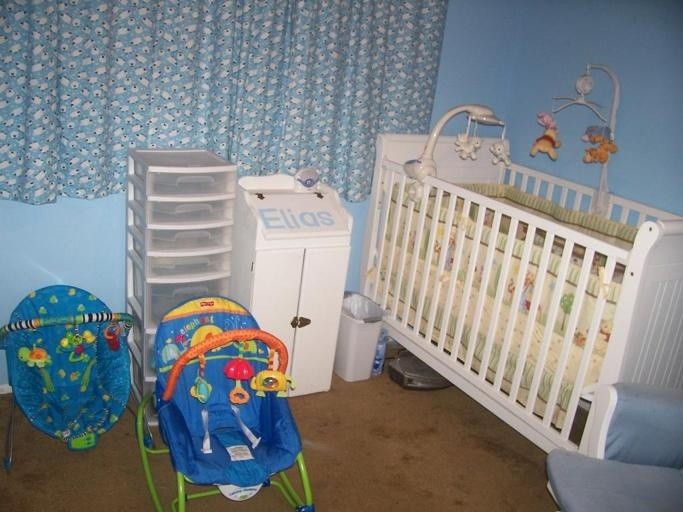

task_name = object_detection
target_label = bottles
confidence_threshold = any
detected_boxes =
[371,324,390,377]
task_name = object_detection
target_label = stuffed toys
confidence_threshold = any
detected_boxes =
[489,142,510,167]
[455,134,483,161]
[581,124,618,164]
[530,111,561,160]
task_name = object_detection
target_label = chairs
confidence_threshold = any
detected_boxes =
[544,381,683,512]
[132,295,314,512]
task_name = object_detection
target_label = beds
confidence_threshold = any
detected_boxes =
[372,129,683,512]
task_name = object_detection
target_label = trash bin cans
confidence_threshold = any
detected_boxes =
[335,293,388,383]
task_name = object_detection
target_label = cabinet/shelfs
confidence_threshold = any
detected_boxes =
[126,143,238,426]
